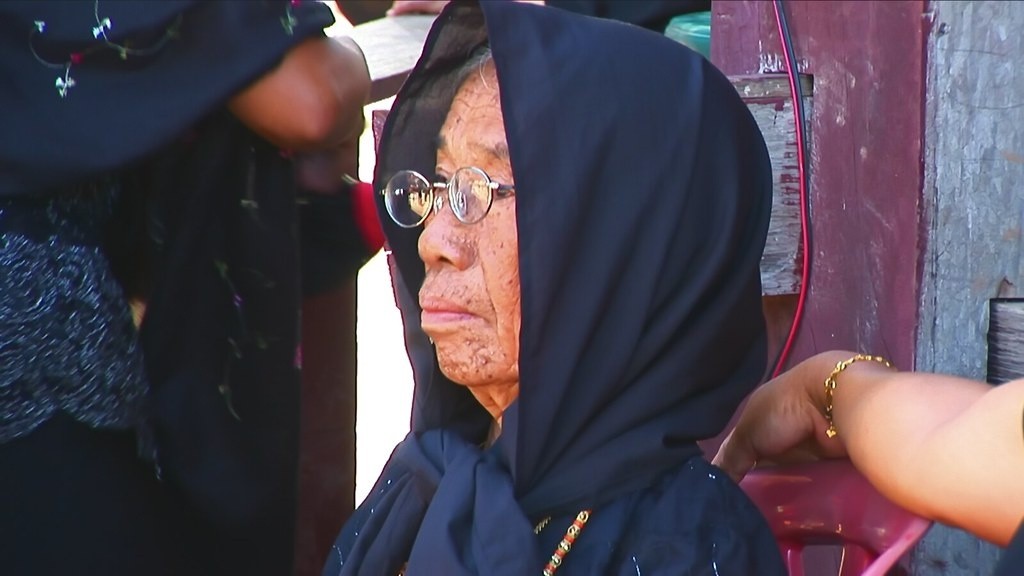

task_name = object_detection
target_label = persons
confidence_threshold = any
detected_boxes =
[320,0,785,576]
[0,0,373,575]
[387,0,710,101]
[709,269,1024,576]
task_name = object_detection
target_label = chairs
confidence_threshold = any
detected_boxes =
[737,455,934,576]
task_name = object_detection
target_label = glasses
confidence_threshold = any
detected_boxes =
[379,166,515,229]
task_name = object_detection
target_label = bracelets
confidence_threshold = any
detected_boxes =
[824,354,892,441]
